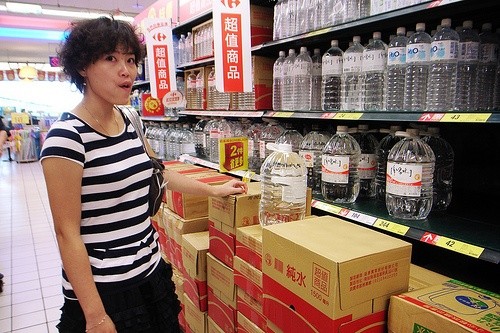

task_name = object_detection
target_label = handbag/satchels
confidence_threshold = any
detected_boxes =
[117,104,166,217]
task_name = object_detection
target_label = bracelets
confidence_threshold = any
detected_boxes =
[86,314,108,331]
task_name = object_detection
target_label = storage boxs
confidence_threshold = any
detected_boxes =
[153,154,500,333]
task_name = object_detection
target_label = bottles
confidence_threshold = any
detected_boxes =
[141,116,454,230]
[273,0,430,41]
[273,19,500,111]
[172,23,255,111]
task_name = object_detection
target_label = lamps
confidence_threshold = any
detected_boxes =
[18,63,38,78]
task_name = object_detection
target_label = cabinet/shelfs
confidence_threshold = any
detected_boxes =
[130,0,500,269]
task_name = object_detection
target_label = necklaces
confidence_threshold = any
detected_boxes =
[82,99,119,136]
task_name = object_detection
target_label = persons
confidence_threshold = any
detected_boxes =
[0,117,11,159]
[40,15,248,333]
[21,109,25,113]
